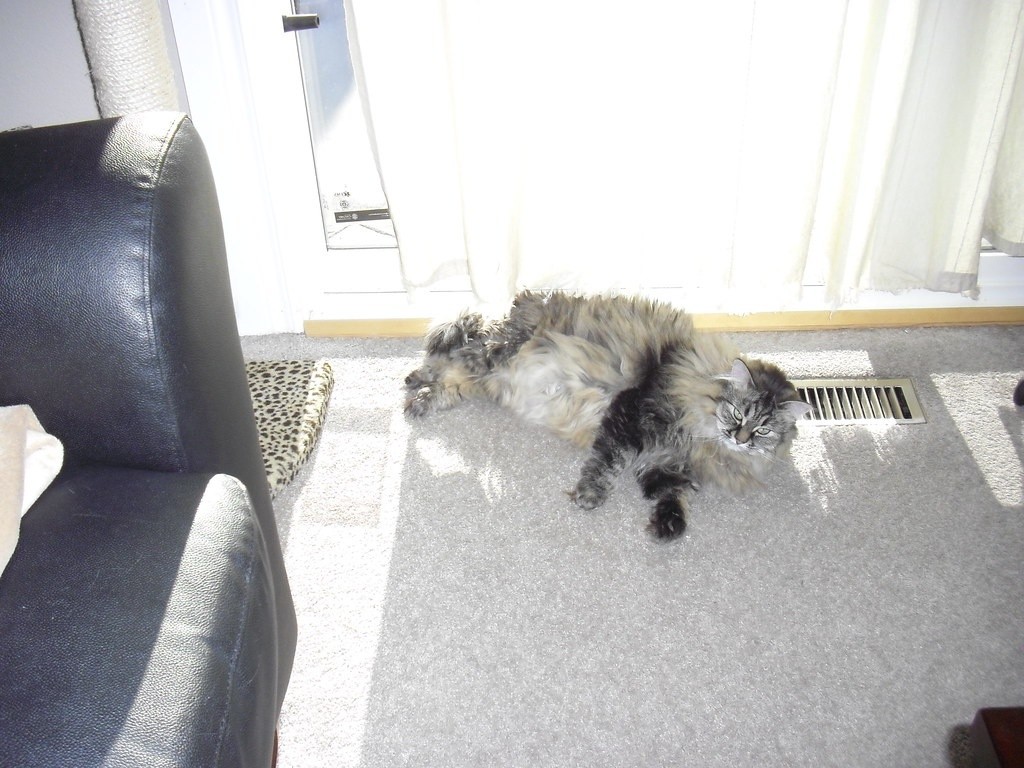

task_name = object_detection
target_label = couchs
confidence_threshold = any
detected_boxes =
[0,116,297,768]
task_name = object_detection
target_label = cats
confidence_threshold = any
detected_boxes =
[401,286,813,546]
